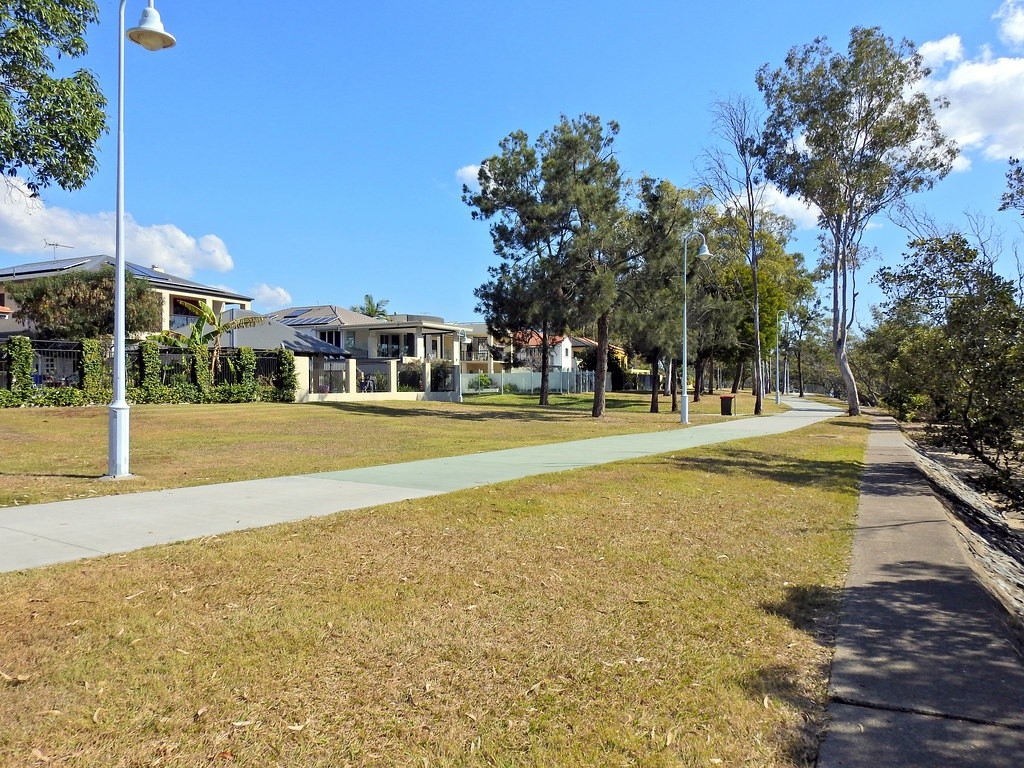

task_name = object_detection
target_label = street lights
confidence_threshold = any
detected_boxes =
[103,0,180,483]
[678,231,715,425]
[775,310,791,405]
